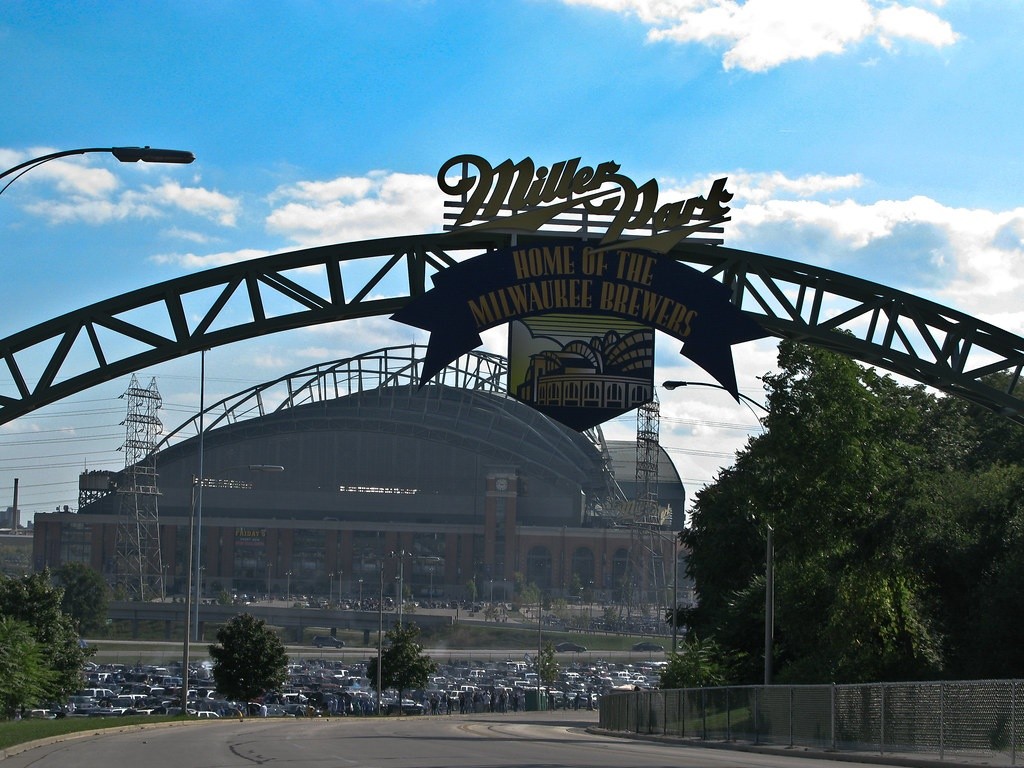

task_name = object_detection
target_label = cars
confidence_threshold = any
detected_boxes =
[555,642,587,653]
[198,593,488,612]
[633,641,665,653]
[30,655,669,719]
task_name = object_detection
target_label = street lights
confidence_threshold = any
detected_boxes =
[199,565,206,599]
[490,578,493,604]
[612,523,678,659]
[285,569,292,607]
[328,569,334,606]
[503,578,507,603]
[338,569,343,606]
[589,578,594,617]
[266,559,273,596]
[162,562,170,599]
[661,379,778,686]
[358,577,364,603]
[473,572,476,604]
[182,464,287,714]
[429,566,433,602]
[390,550,413,632]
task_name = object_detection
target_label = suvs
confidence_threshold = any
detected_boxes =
[312,635,346,650]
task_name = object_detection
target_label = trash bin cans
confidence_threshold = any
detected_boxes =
[525,688,548,711]
[549,690,566,709]
